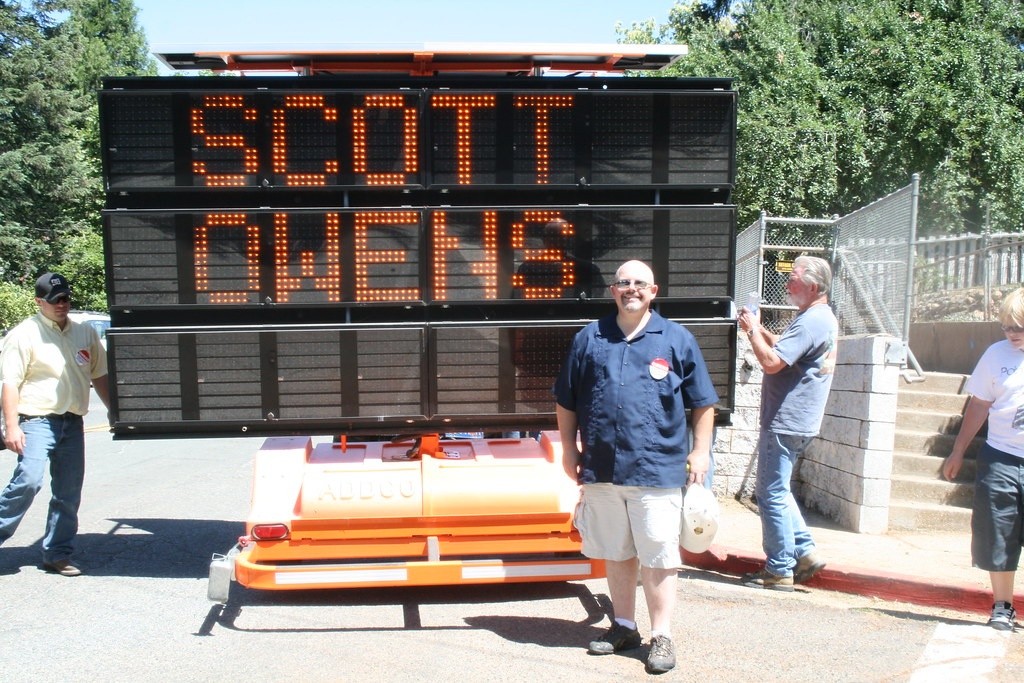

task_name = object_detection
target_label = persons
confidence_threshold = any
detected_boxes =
[0,273,116,576]
[554,259,719,673]
[944,286,1024,630]
[737,256,839,592]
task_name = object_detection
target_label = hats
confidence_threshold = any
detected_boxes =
[35,273,73,301]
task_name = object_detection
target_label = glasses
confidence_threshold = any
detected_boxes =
[1000,326,1024,332]
[45,294,71,304]
[613,279,652,289]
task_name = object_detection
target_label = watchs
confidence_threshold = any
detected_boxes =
[746,329,755,338]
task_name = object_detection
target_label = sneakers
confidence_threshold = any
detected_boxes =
[50,559,82,576]
[986,601,1016,630]
[589,622,641,654]
[647,636,676,671]
[742,569,794,592]
[793,553,826,582]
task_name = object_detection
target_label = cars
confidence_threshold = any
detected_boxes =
[66,309,111,343]
